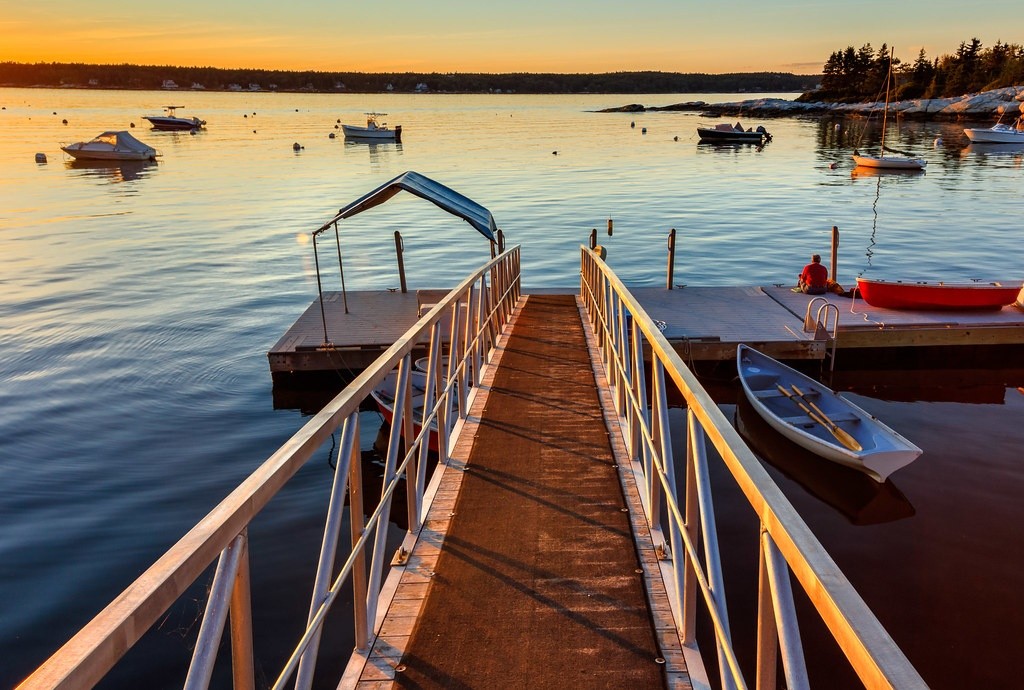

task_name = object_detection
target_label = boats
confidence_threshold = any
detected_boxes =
[141,107,206,131]
[61,130,163,160]
[963,123,1024,144]
[737,343,924,483]
[696,124,763,143]
[68,158,158,182]
[369,354,484,453]
[341,113,401,138]
[854,275,1024,310]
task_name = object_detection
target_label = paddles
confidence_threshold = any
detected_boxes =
[374,390,437,425]
[773,380,864,452]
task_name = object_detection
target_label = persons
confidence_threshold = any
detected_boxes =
[797,255,828,296]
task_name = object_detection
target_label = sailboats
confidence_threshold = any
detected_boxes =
[851,46,928,169]
